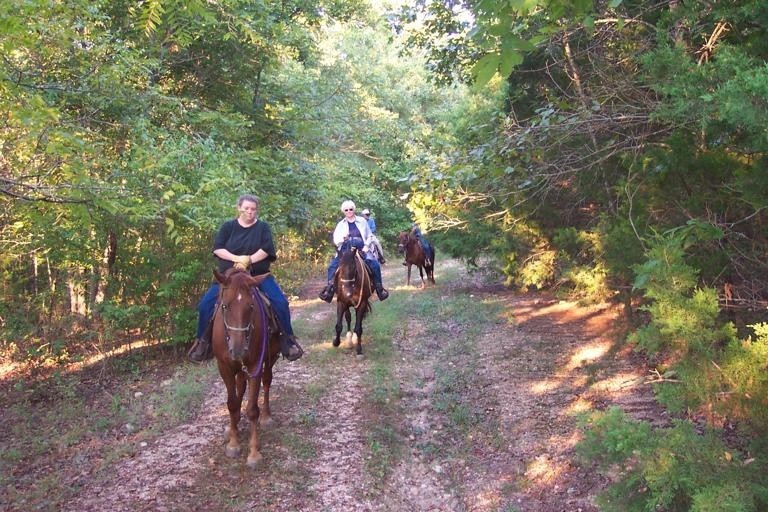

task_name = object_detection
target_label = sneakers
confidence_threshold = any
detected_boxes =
[279,331,304,362]
[322,285,335,304]
[190,339,214,361]
[376,287,389,301]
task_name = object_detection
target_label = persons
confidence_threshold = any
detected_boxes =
[190,193,302,364]
[320,199,389,302]
[402,220,433,266]
[361,209,386,264]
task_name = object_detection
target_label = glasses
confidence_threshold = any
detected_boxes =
[345,208,353,211]
[363,213,370,215]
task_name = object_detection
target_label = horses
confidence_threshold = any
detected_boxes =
[333,247,376,362]
[398,229,435,290]
[212,268,281,469]
